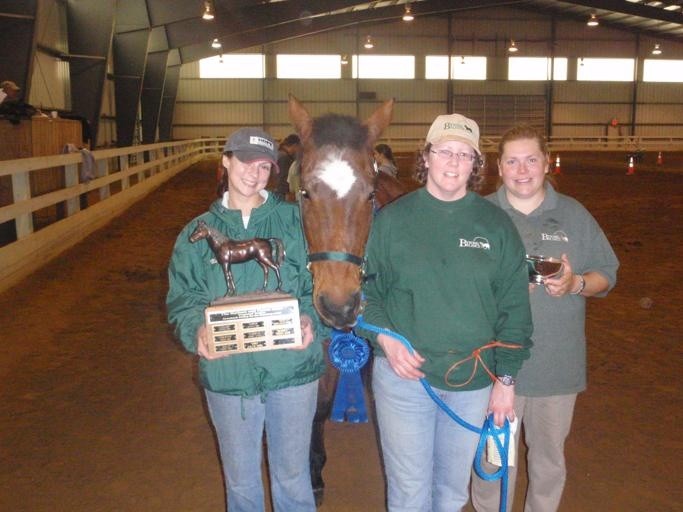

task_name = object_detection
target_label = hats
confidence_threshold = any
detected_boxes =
[423,113,480,156]
[223,129,281,174]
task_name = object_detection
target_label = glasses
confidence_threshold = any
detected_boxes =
[429,148,478,165]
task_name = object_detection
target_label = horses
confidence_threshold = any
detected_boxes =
[188,219,285,298]
[283,92,423,508]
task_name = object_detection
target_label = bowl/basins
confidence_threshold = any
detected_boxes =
[525,255,564,286]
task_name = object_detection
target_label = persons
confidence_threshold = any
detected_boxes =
[469,120,621,512]
[372,143,398,179]
[350,111,534,511]
[271,131,303,200]
[160,124,334,511]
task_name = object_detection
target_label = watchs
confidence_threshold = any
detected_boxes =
[567,272,586,295]
[497,374,515,387]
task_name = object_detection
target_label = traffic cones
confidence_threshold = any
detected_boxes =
[552,153,562,175]
[624,156,637,175]
[653,152,665,167]
[215,152,227,190]
